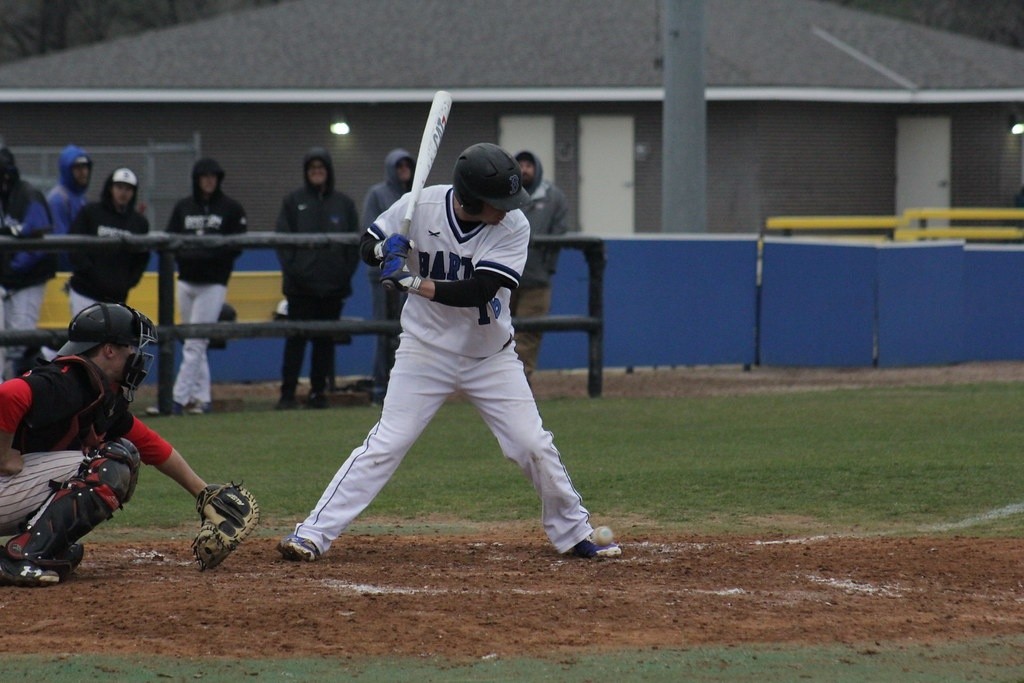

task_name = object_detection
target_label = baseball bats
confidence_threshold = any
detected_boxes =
[381,89,453,287]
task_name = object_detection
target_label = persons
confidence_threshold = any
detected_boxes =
[45,145,93,272]
[508,149,570,387]
[275,141,622,563]
[63,167,150,322]
[272,147,359,411]
[361,149,417,405]
[0,142,53,387]
[0,300,261,585]
[147,157,247,416]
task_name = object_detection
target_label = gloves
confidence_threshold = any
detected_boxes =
[372,259,415,292]
[378,233,416,259]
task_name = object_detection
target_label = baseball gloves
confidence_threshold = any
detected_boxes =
[195,481,260,574]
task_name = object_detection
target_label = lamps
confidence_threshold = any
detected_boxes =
[330,111,349,134]
[1009,112,1024,135]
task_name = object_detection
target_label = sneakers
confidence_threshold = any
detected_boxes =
[275,531,319,563]
[0,544,60,588]
[572,529,622,562]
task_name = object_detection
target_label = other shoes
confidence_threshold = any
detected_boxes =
[306,388,329,409]
[276,383,297,410]
[142,399,184,415]
[189,401,214,415]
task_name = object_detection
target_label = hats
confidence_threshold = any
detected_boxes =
[55,300,159,357]
[111,167,138,187]
[451,141,532,215]
